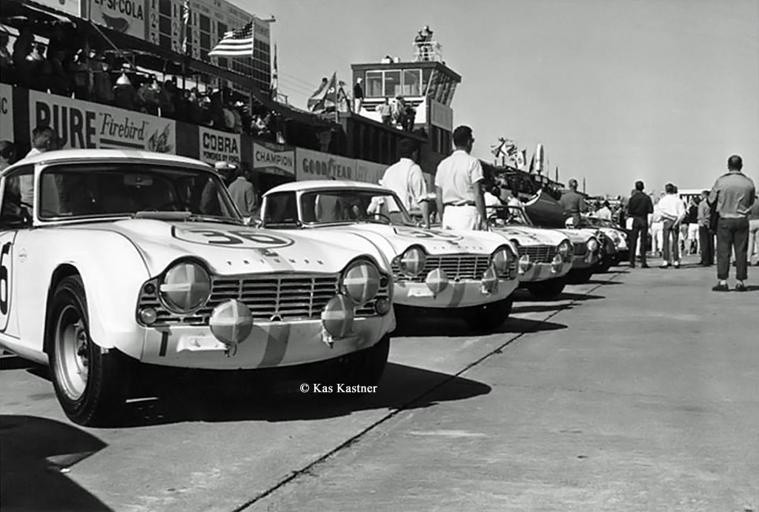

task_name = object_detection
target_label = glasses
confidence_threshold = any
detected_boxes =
[468,138,474,142]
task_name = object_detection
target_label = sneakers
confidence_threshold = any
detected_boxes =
[732,260,759,268]
[660,261,681,269]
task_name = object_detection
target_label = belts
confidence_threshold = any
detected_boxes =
[443,202,476,207]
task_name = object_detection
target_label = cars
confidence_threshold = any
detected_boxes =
[257,178,519,323]
[0,149,397,425]
[483,205,651,296]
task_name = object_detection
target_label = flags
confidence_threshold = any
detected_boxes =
[268,47,278,97]
[307,69,350,112]
[207,19,255,57]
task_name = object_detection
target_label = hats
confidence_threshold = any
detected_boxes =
[214,161,236,169]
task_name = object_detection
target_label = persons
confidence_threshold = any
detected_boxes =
[1,30,252,134]
[559,154,759,291]
[434,125,489,229]
[376,93,417,131]
[483,182,528,226]
[222,159,259,218]
[199,161,237,216]
[352,75,366,113]
[0,141,27,218]
[367,137,431,227]
[415,24,435,60]
[17,125,60,218]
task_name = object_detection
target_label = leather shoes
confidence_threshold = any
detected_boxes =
[735,283,744,290]
[712,281,729,291]
[697,261,714,267]
[628,263,635,268]
[640,264,649,267]
